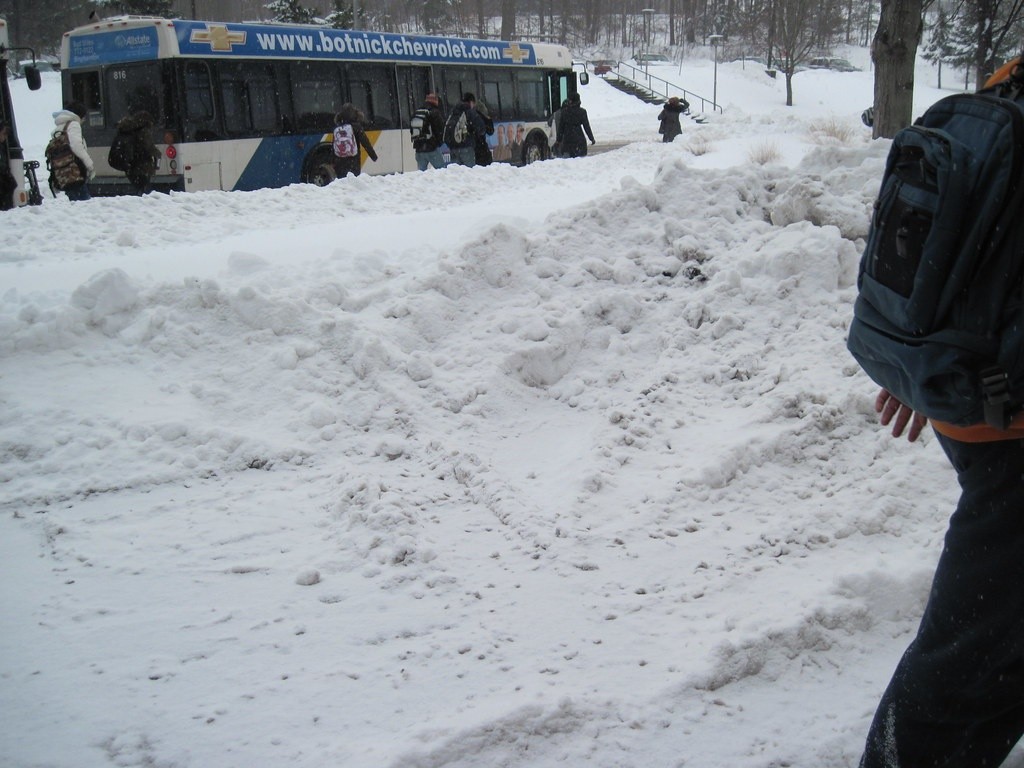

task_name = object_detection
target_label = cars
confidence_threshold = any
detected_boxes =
[16,60,56,79]
[740,57,809,74]
[632,54,676,67]
[5,68,17,80]
[584,59,619,73]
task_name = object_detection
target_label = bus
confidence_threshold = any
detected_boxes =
[58,12,591,203]
[1,44,42,213]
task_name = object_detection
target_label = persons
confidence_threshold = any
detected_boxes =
[493,123,525,163]
[658,97,690,142]
[841,34,1024,768]
[551,92,595,158]
[44,102,96,202]
[332,102,379,179]
[443,92,494,168]
[410,92,447,171]
[0,118,18,211]
[108,111,162,197]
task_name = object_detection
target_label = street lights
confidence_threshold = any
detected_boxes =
[644,7,654,78]
[710,35,723,112]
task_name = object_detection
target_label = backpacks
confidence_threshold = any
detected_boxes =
[333,123,359,158]
[410,106,439,141]
[45,121,88,198]
[108,132,146,171]
[845,87,1023,431]
[443,109,475,149]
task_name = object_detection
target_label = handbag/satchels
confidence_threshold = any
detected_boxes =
[548,120,556,146]
[659,120,664,134]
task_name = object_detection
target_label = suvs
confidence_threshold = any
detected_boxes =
[808,58,863,72]
